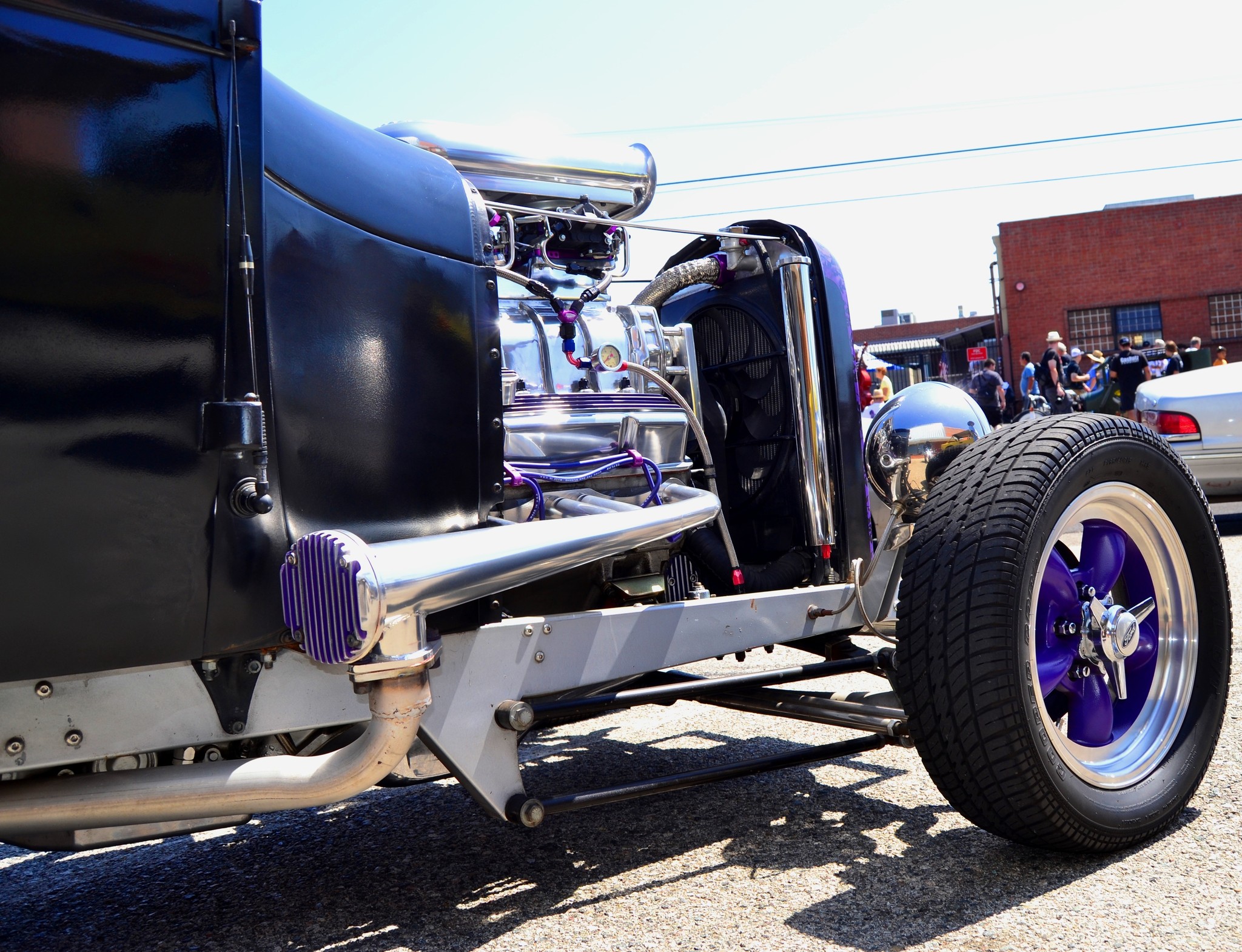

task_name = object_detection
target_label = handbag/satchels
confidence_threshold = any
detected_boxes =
[977,373,997,401]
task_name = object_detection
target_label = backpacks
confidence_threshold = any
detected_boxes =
[1034,348,1061,388]
[1060,362,1078,388]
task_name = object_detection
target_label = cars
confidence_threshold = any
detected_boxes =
[0,2,1237,855]
[1134,361,1242,504]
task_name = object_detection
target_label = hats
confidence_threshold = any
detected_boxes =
[1143,341,1150,347]
[1046,331,1063,342]
[870,389,886,398]
[1120,337,1130,345]
[1071,348,1085,358]
[1155,339,1166,348]
[967,421,974,426]
[1086,350,1105,364]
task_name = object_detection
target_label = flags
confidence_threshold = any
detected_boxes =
[938,351,949,383]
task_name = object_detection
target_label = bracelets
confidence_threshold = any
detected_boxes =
[976,436,978,437]
[1027,389,1032,393]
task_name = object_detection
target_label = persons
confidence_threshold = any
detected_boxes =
[1020,331,1201,422]
[860,362,894,418]
[923,439,932,463]
[969,358,1016,430]
[1213,347,1228,366]
[967,420,980,442]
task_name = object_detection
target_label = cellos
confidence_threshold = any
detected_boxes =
[856,341,872,413]
